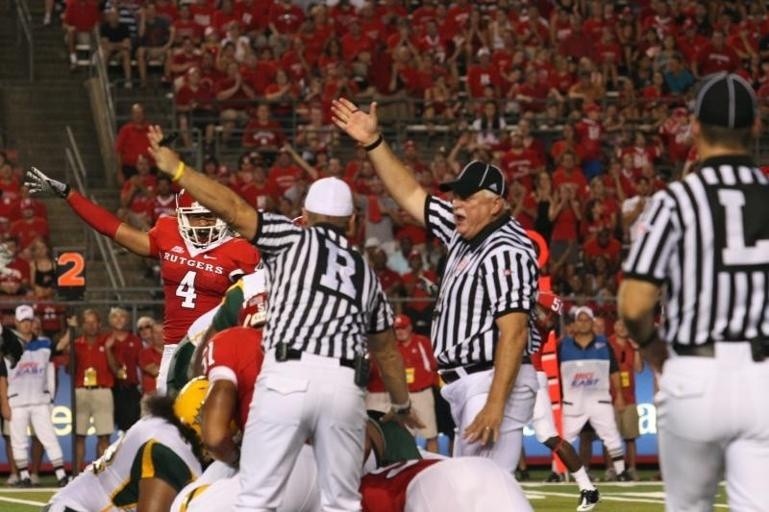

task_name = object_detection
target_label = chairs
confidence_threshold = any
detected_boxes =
[6,474,68,487]
[522,468,640,512]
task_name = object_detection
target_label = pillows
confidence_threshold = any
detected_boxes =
[176,187,229,246]
[239,293,268,328]
[171,376,238,447]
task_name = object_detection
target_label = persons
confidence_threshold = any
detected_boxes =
[0,0,767,511]
[330,96,541,483]
[144,126,427,508]
[616,70,766,512]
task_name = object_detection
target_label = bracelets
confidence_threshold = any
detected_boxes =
[638,322,658,346]
[364,133,383,151]
[390,399,411,414]
[171,161,184,181]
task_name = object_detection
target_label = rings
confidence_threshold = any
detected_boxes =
[486,426,490,433]
[352,108,360,113]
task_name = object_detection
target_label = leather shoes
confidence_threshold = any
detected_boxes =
[24,167,70,199]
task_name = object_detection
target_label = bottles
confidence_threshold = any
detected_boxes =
[695,72,756,129]
[15,305,34,322]
[304,176,353,217]
[575,306,593,320]
[393,315,410,328]
[438,161,508,199]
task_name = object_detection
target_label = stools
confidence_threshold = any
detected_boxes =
[441,356,531,384]
[286,350,355,368]
[672,339,769,358]
